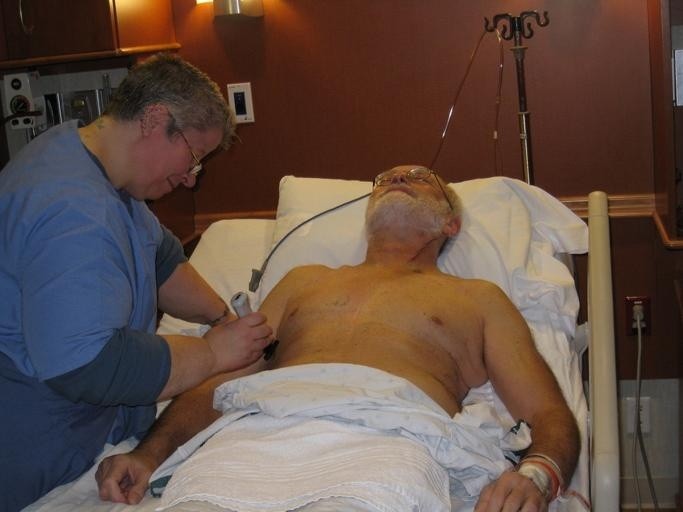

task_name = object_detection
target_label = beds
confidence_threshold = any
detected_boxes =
[16,185,623,512]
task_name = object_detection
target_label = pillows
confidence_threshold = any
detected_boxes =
[253,174,532,337]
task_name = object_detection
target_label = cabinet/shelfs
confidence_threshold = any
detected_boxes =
[0,0,182,75]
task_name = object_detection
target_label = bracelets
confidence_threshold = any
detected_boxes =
[209,301,230,327]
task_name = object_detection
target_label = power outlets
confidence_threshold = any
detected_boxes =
[621,395,651,436]
[625,295,652,335]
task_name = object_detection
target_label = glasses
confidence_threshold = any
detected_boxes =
[374,166,455,212]
[168,109,202,177]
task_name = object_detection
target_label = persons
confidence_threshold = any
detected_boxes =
[93,163,581,512]
[0,45,275,512]
